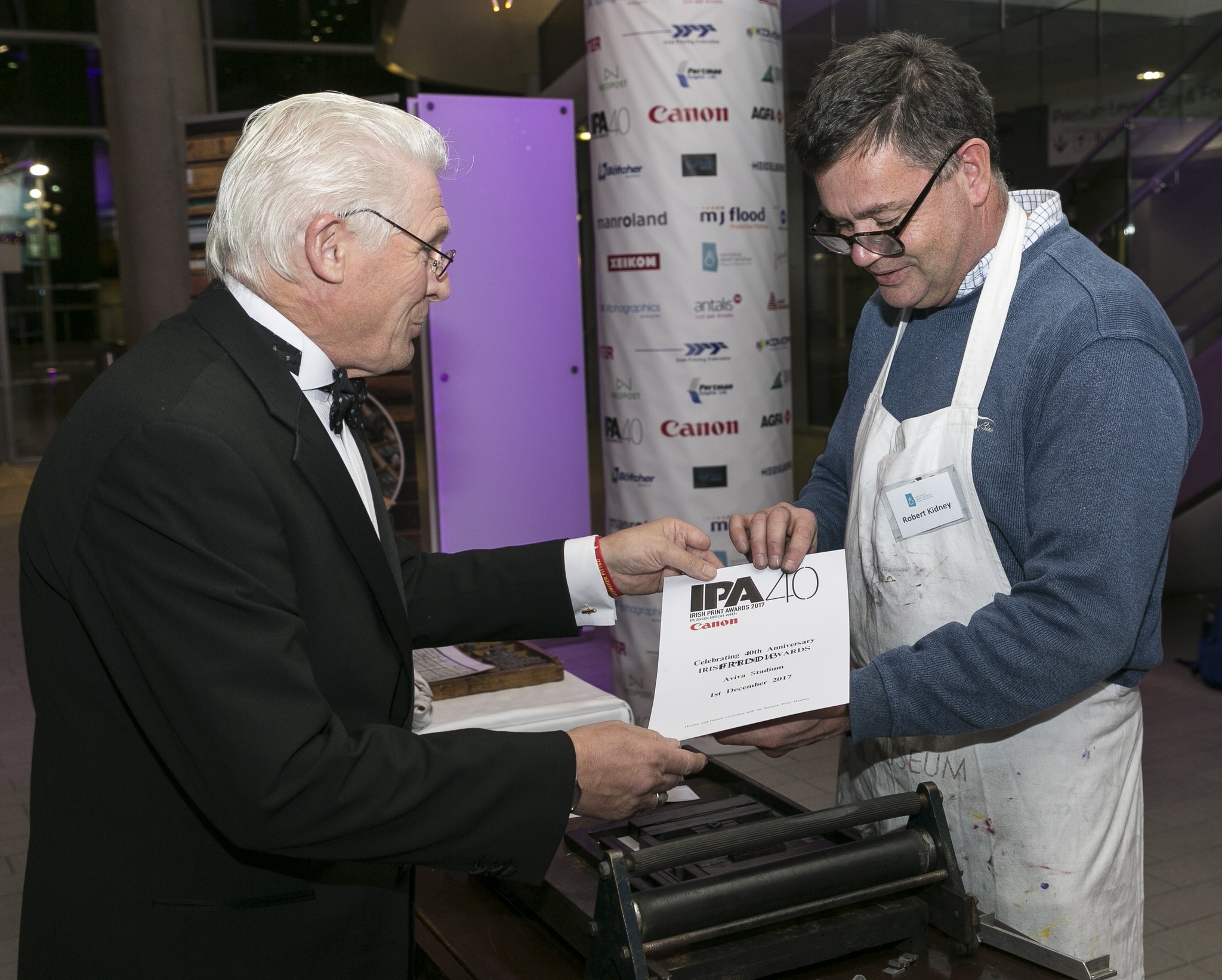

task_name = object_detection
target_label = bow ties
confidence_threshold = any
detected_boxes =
[254,320,369,439]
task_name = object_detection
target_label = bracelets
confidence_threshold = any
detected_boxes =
[593,536,623,600]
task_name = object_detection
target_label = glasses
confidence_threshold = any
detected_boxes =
[809,140,970,257]
[340,209,456,278]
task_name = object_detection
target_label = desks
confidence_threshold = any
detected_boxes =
[413,777,1066,980]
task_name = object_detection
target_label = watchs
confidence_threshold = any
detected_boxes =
[571,776,582,812]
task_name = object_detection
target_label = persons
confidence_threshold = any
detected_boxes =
[17,93,725,980]
[708,31,1200,980]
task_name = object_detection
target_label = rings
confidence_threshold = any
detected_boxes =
[647,792,662,811]
